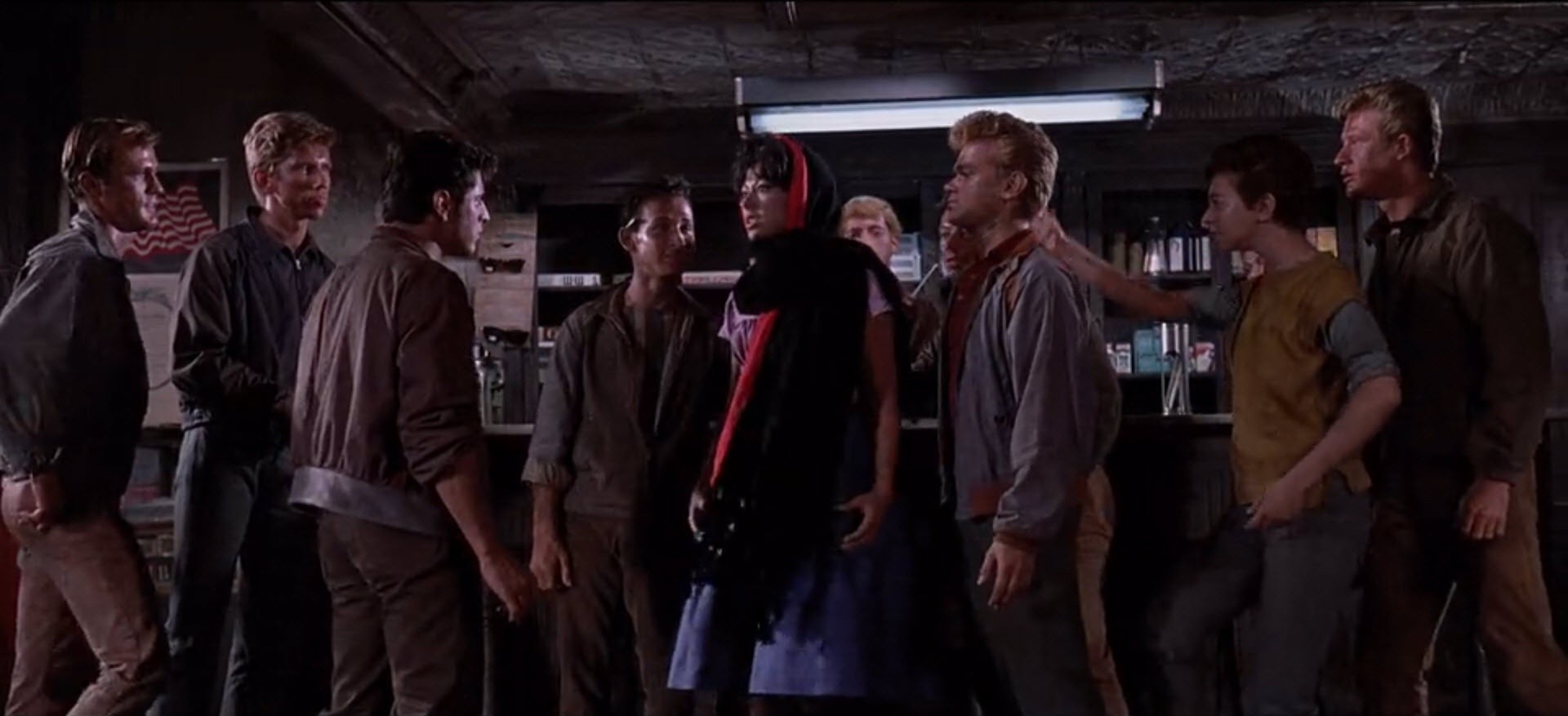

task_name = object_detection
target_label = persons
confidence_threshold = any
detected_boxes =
[520,176,730,716]
[290,124,538,716]
[144,111,339,716]
[1042,127,1401,716]
[656,134,922,715]
[908,112,1130,716]
[0,119,164,716]
[1333,76,1568,716]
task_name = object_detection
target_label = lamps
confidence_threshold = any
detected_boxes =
[732,59,1166,141]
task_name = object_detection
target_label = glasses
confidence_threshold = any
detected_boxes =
[480,258,525,273]
[485,324,529,349]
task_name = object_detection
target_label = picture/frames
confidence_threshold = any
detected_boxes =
[60,163,230,279]
